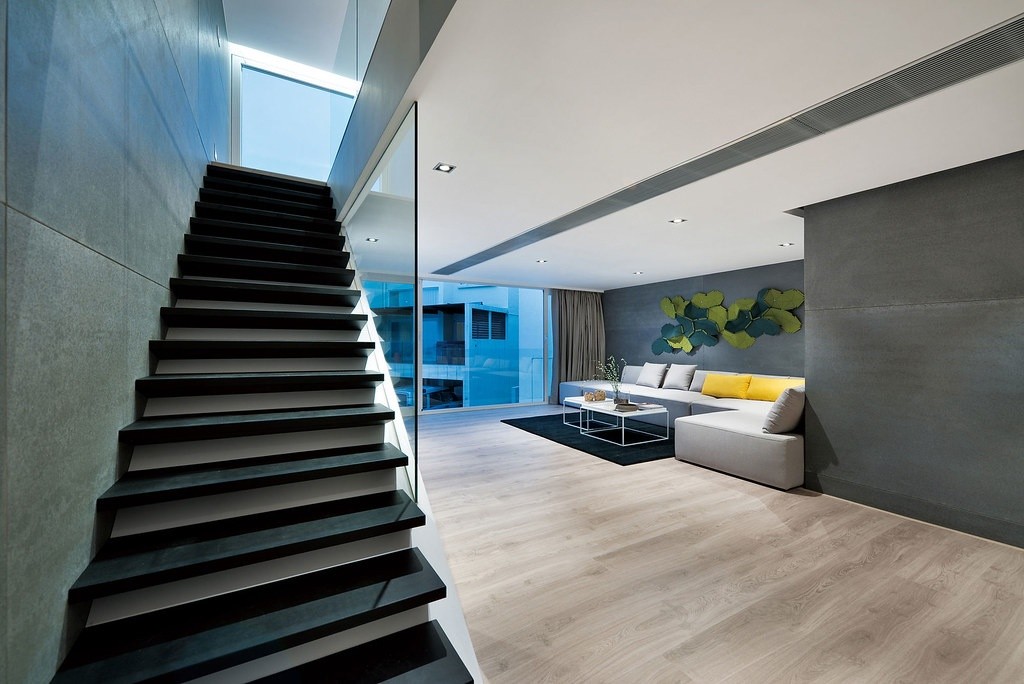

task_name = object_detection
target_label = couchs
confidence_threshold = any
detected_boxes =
[559,362,805,490]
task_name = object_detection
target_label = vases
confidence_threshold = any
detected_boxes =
[611,384,628,403]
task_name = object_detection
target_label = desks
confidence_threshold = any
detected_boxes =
[394,385,446,410]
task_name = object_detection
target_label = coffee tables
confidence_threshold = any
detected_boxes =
[563,396,670,447]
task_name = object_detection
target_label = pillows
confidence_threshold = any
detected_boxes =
[689,370,737,392]
[662,364,698,391]
[636,362,667,388]
[761,385,805,433]
[701,373,752,398]
[744,377,804,402]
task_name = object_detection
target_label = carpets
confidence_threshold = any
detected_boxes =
[500,411,675,466]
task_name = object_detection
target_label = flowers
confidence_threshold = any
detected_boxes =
[577,356,628,392]
